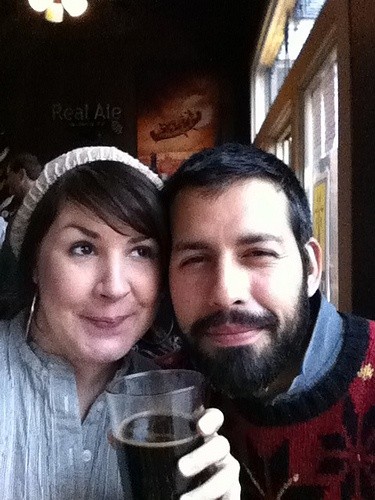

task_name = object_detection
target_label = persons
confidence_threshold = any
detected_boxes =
[0,147,241,500]
[1,151,42,320]
[152,144,375,500]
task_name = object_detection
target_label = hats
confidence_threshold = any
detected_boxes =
[9,145,167,261]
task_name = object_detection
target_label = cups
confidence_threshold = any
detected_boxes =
[106,368,208,500]
